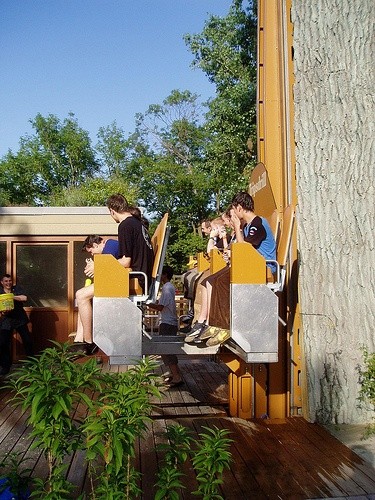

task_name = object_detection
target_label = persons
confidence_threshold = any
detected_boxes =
[0,274,38,377]
[180,191,276,347]
[148,265,185,389]
[66,193,155,356]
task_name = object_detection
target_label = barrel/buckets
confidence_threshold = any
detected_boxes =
[0,293,15,311]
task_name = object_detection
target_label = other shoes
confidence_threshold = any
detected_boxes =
[206,328,230,347]
[68,342,85,354]
[179,315,208,343]
[1,374,16,382]
[161,368,172,379]
[82,341,99,355]
[165,378,185,387]
[194,325,222,343]
